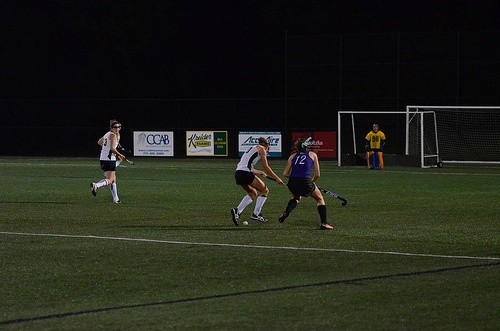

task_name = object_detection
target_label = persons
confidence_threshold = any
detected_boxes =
[90,120,127,205]
[363,122,386,171]
[230,137,283,226]
[277,138,334,230]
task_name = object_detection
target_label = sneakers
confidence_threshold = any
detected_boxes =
[112,198,121,203]
[320,223,333,230]
[231,208,240,226]
[250,213,269,222]
[90,183,97,196]
[279,213,288,223]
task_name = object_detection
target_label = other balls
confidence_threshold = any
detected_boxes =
[243,221,248,225]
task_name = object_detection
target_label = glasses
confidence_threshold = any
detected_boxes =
[112,126,120,128]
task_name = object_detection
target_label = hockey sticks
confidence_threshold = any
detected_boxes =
[261,174,288,186]
[316,186,348,206]
[116,151,135,165]
